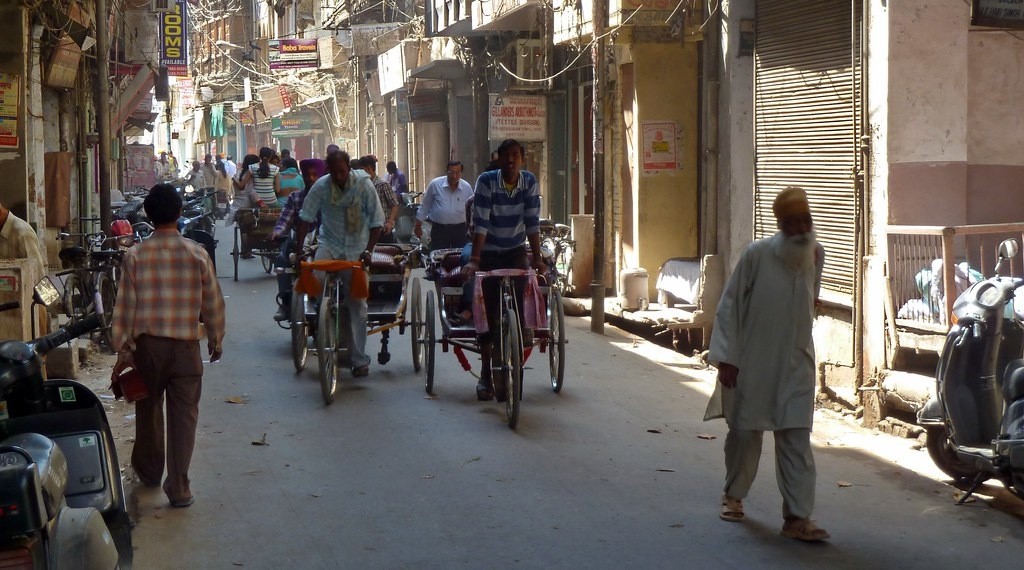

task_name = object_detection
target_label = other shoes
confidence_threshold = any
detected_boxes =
[456,315,468,325]
[521,326,534,346]
[476,379,494,402]
[172,497,195,507]
[353,365,369,377]
[273,304,289,322]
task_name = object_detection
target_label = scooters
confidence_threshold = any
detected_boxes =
[0,276,134,570]
[916,238,1024,505]
[215,189,230,219]
[395,192,424,244]
[112,185,220,276]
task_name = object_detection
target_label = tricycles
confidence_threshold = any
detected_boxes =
[276,235,424,404]
[229,207,284,281]
[418,248,569,430]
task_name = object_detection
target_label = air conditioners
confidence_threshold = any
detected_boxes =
[502,39,544,85]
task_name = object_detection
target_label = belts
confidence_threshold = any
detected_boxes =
[438,222,466,227]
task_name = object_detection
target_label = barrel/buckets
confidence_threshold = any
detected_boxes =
[620,268,649,311]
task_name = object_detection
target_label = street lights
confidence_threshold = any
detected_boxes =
[217,40,259,155]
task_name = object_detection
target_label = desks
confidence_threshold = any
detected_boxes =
[655,260,700,308]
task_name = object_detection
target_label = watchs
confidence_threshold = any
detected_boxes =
[533,252,543,257]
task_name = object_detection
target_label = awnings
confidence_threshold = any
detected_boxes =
[185,100,254,121]
[121,111,159,132]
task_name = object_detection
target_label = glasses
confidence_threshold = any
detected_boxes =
[447,170,463,175]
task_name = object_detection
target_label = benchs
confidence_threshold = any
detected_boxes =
[369,242,420,283]
[253,207,282,228]
[432,249,551,295]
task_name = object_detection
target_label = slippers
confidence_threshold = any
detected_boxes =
[720,492,744,522]
[783,516,828,540]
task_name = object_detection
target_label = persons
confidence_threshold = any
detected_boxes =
[413,160,474,250]
[152,152,178,182]
[107,183,226,507]
[188,154,237,220]
[703,188,833,541]
[385,161,409,202]
[225,146,305,260]
[0,202,49,382]
[463,139,547,401]
[270,144,386,377]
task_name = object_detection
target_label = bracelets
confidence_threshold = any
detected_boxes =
[364,250,373,257]
[256,199,264,205]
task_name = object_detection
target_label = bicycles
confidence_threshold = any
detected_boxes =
[55,222,155,352]
[538,218,578,296]
